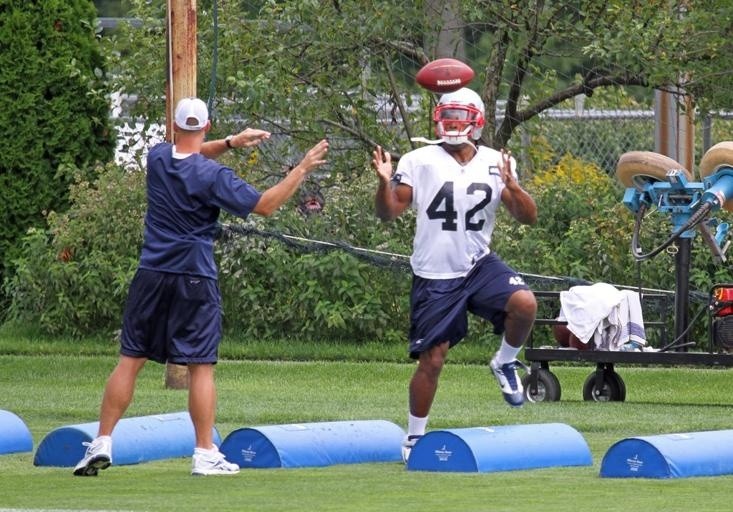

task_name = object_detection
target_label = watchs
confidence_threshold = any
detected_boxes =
[225,134,235,149]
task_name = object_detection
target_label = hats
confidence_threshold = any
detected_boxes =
[175,97,209,131]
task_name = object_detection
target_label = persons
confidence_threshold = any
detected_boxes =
[71,95,329,478]
[371,86,538,466]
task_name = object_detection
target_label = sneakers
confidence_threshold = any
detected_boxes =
[72,436,113,476]
[191,445,240,475]
[401,434,423,464]
[490,352,526,406]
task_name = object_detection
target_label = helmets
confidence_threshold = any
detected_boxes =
[433,87,487,145]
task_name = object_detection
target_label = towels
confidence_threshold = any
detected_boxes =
[593,288,647,352]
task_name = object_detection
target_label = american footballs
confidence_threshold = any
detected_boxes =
[569,333,593,350]
[554,317,570,347]
[416,58,474,93]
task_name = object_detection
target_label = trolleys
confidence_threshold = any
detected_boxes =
[524,140,733,402]
